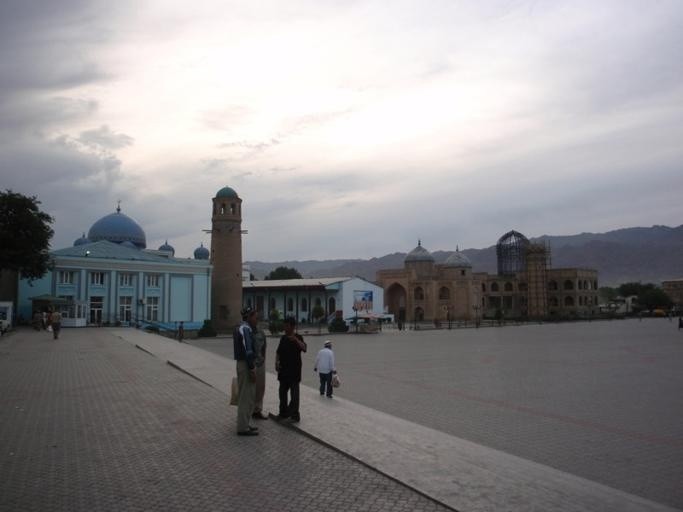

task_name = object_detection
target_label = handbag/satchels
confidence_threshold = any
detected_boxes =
[230,376,240,406]
[330,373,341,388]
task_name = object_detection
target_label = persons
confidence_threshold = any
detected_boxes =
[178,320,185,343]
[678,317,683,330]
[233,306,261,438]
[313,339,336,398]
[32,306,62,339]
[276,314,306,421]
[252,322,270,421]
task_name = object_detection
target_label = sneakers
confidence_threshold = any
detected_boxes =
[320,392,324,395]
[276,414,298,423]
[326,394,332,398]
[248,425,259,431]
[252,413,269,420]
[237,430,259,436]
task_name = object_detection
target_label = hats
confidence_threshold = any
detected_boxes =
[324,340,333,346]
[240,306,257,322]
